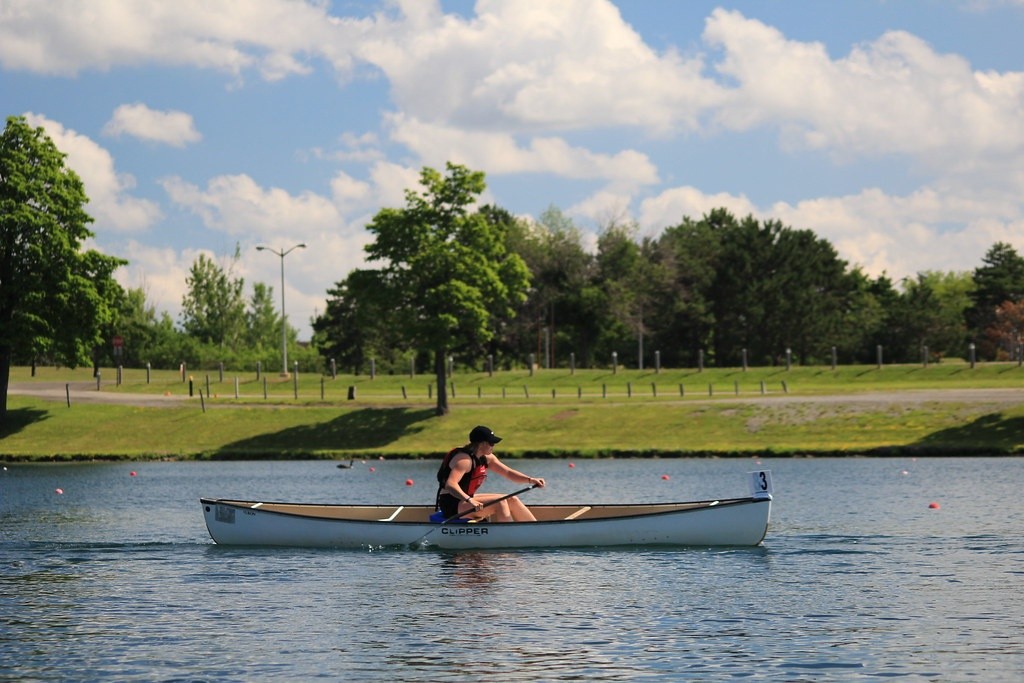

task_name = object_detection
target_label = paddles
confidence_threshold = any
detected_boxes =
[408,480,542,546]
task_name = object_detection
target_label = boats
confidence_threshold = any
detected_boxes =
[199,469,772,549]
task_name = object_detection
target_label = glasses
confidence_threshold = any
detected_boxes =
[482,441,494,446]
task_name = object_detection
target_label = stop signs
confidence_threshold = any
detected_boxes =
[113,337,122,347]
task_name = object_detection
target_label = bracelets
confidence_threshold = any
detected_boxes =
[529,478,530,482]
[466,497,471,502]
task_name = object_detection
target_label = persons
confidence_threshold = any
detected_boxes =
[438,426,545,521]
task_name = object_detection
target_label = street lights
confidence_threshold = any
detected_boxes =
[256,242,306,374]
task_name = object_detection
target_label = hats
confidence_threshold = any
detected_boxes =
[469,426,502,443]
